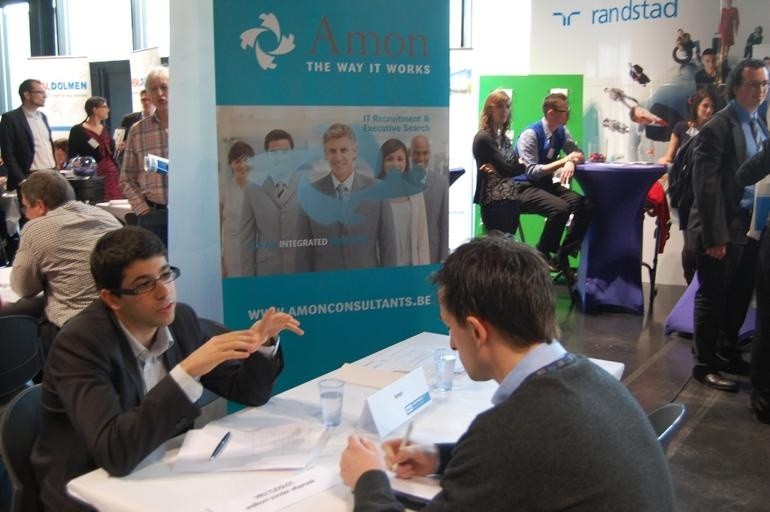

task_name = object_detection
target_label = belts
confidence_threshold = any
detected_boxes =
[144,198,167,210]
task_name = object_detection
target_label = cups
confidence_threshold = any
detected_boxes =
[435,354,456,393]
[317,380,347,426]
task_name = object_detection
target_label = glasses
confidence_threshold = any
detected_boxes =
[554,107,571,113]
[107,265,182,296]
[739,81,770,89]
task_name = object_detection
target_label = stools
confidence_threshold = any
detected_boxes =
[481,207,533,243]
[450,168,465,187]
[640,181,672,315]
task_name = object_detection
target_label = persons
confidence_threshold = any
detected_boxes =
[603,1,765,143]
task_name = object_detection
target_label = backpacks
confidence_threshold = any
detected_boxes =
[668,132,696,209]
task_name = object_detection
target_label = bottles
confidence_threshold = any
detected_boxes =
[71,155,98,179]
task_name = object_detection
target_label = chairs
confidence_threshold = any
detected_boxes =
[647,402,688,454]
[1,315,42,408]
[1,382,42,511]
[125,212,167,251]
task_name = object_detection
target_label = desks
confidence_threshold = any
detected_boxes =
[574,160,667,317]
[0,170,140,315]
[35,331,625,511]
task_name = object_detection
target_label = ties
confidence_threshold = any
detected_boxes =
[336,185,348,226]
[276,180,286,199]
[655,120,670,126]
[749,120,764,154]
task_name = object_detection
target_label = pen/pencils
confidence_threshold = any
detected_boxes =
[395,421,413,480]
[210,432,230,460]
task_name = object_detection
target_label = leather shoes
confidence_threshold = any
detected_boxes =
[536,245,560,273]
[554,249,575,278]
[721,359,751,376]
[699,371,740,391]
